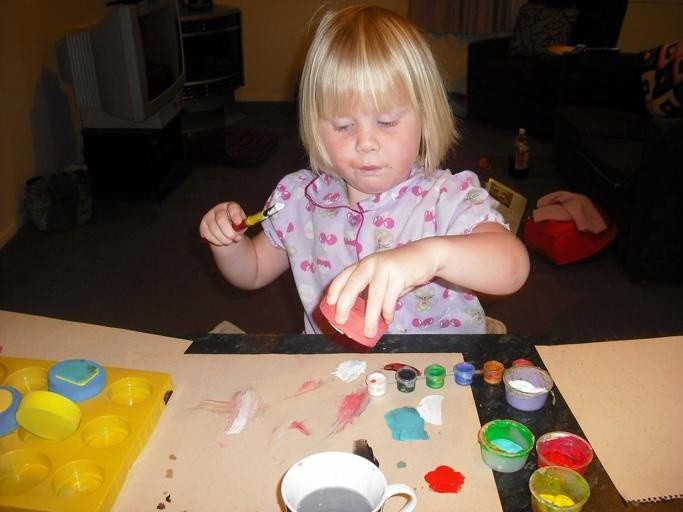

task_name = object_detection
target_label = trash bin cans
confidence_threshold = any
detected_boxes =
[27,177,70,232]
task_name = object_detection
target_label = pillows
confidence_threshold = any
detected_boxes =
[522,199,618,267]
[638,38,682,118]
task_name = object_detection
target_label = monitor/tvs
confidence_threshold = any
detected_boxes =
[52,0,187,124]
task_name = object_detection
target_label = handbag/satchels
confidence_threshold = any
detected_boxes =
[25,170,93,232]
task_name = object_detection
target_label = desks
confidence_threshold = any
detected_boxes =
[80,88,234,210]
[186,333,683,512]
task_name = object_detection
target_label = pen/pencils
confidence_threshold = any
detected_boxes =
[196,204,283,244]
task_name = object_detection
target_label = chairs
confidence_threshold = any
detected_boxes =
[467,0,582,121]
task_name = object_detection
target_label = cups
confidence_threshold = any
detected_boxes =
[276,450,418,511]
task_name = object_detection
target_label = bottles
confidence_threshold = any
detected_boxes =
[513,129,530,178]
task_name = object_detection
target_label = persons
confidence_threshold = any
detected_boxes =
[196,4,533,343]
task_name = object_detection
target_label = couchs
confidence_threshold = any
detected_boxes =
[535,48,682,283]
